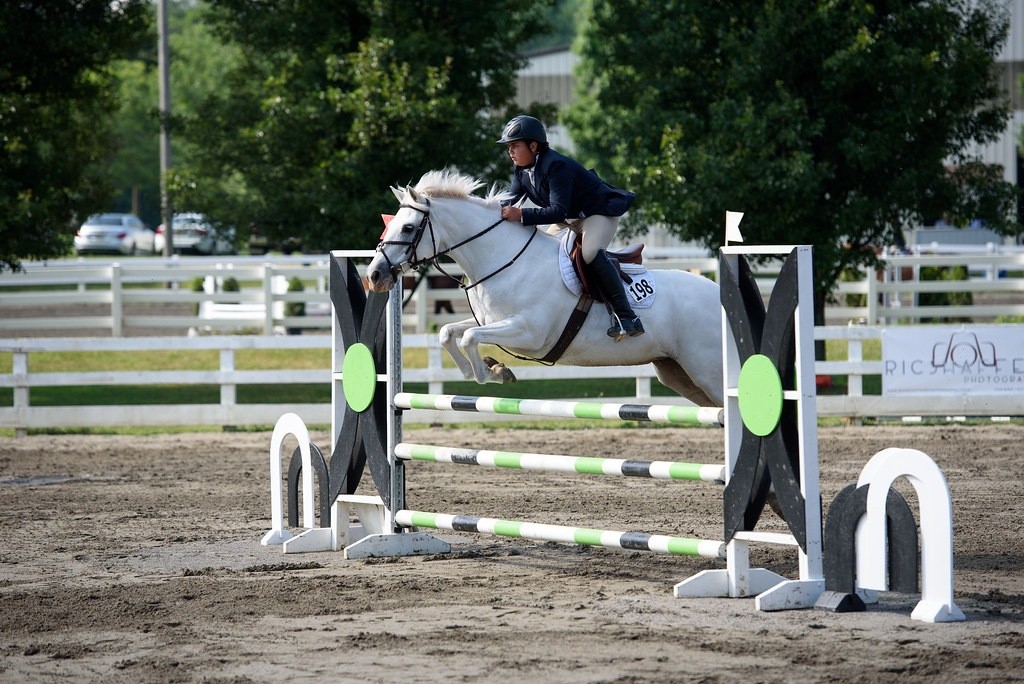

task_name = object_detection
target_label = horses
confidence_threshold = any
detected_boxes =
[365,163,724,407]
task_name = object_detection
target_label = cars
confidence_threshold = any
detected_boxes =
[73,210,156,256]
[151,212,236,257]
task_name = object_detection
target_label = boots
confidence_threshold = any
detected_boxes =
[586,249,645,338]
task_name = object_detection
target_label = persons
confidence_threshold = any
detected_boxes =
[496,115,645,337]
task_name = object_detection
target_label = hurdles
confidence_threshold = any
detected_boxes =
[261,209,965,623]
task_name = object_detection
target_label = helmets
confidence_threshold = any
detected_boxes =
[495,115,546,144]
